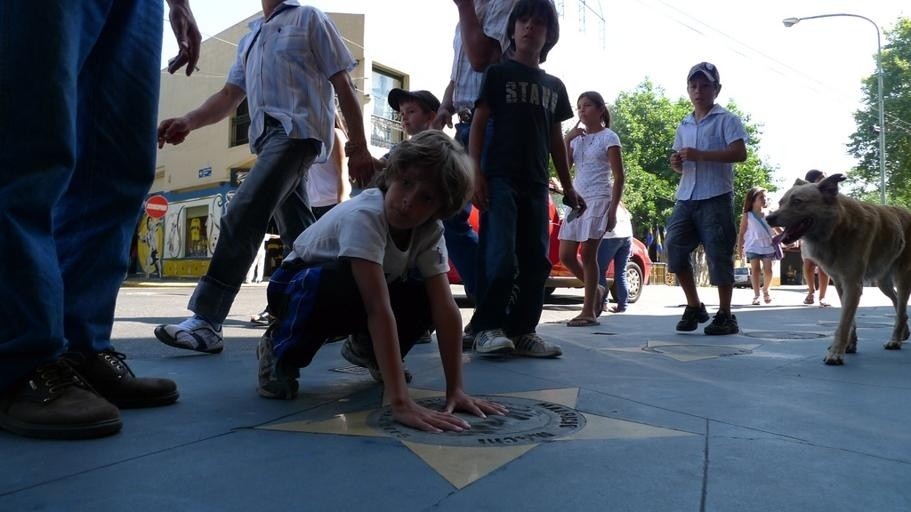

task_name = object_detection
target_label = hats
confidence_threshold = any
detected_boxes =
[688,62,719,85]
[388,88,440,113]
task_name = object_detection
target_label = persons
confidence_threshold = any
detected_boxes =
[345,90,478,304]
[737,186,798,305]
[0,0,205,442]
[789,170,832,308]
[151,0,378,354]
[257,130,513,433]
[439,0,586,357]
[250,108,346,326]
[556,92,634,326]
[667,62,748,335]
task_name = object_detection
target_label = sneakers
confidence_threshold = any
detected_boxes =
[341,332,412,383]
[0,346,179,441]
[704,311,739,335]
[462,323,561,356]
[154,313,223,354]
[676,303,709,331]
[251,309,300,399]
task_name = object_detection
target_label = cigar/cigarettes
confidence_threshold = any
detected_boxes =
[179,47,202,73]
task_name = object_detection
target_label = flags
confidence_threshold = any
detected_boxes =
[640,217,668,263]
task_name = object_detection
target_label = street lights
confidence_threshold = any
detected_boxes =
[784,13,888,205]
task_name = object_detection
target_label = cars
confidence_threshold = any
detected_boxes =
[445,170,653,304]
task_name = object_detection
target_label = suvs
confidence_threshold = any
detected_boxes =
[733,267,764,288]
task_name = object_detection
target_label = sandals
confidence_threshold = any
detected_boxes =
[753,286,772,305]
[804,289,831,307]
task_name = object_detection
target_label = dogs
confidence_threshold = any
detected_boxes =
[765,173,911,366]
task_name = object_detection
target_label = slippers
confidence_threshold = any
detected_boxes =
[567,287,627,326]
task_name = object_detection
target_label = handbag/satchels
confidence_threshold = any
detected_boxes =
[768,236,784,260]
